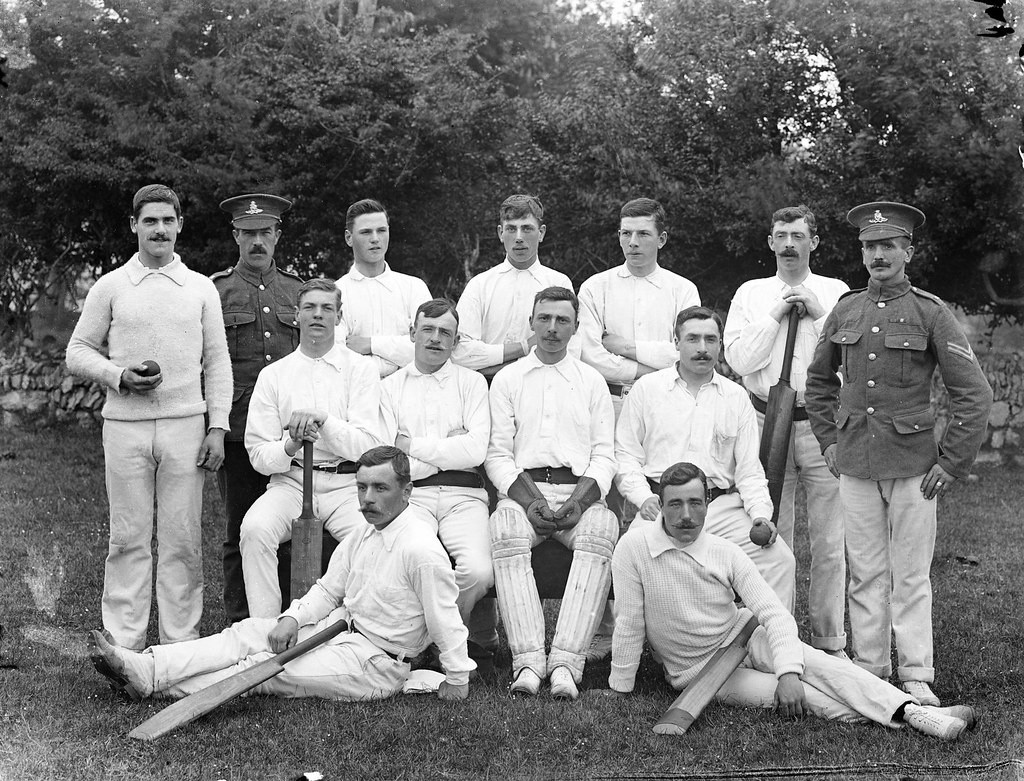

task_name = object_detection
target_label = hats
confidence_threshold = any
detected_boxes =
[847,202,925,241]
[220,194,292,230]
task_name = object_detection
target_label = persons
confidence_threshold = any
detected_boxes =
[239,278,384,619]
[381,298,494,676]
[447,194,581,660]
[86,447,472,704]
[722,207,852,663]
[489,286,620,698]
[331,199,434,384]
[201,193,308,625]
[577,197,702,663]
[65,184,235,649]
[803,201,994,707]
[589,463,976,742]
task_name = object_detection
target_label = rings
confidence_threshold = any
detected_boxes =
[937,482,942,486]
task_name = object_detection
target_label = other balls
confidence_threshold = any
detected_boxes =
[138,358,163,375]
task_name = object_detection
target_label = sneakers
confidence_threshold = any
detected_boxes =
[920,705,977,731]
[903,681,940,706]
[550,666,578,700]
[87,630,154,704]
[903,703,967,739]
[511,668,541,695]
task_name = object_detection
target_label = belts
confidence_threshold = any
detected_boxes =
[607,384,622,396]
[750,391,809,421]
[645,476,726,503]
[412,470,483,488]
[291,461,357,473]
[354,628,413,663]
[524,467,581,484]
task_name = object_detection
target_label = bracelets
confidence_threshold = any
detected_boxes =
[520,339,530,356]
